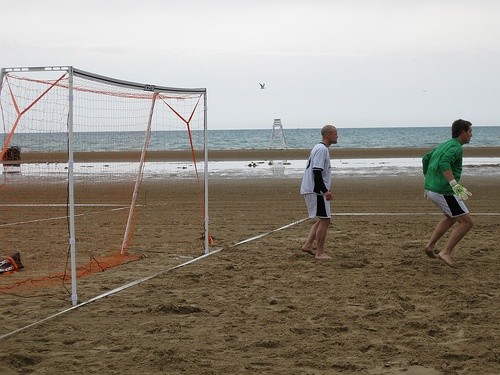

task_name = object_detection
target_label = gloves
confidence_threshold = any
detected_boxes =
[450,179,472,200]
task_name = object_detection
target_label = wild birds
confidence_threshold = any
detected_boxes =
[259,83,265,90]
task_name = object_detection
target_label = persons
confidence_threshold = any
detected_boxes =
[300,124,339,260]
[421,119,474,270]
